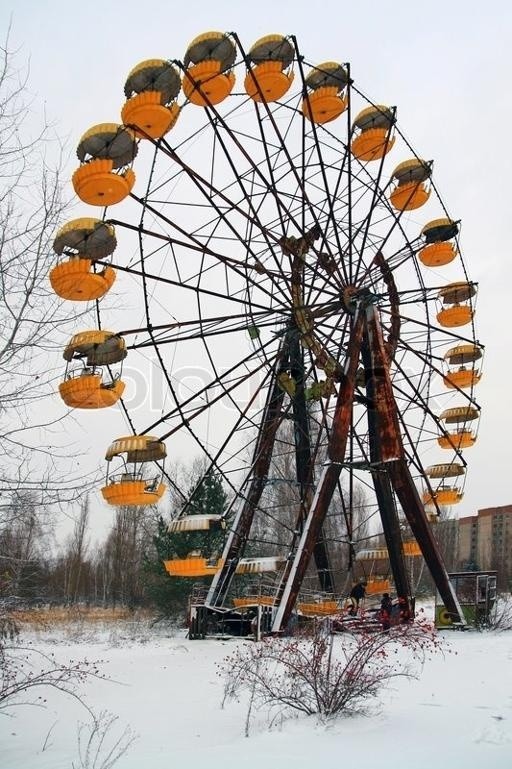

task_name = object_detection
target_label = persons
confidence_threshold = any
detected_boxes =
[380,593,392,634]
[350,581,368,617]
